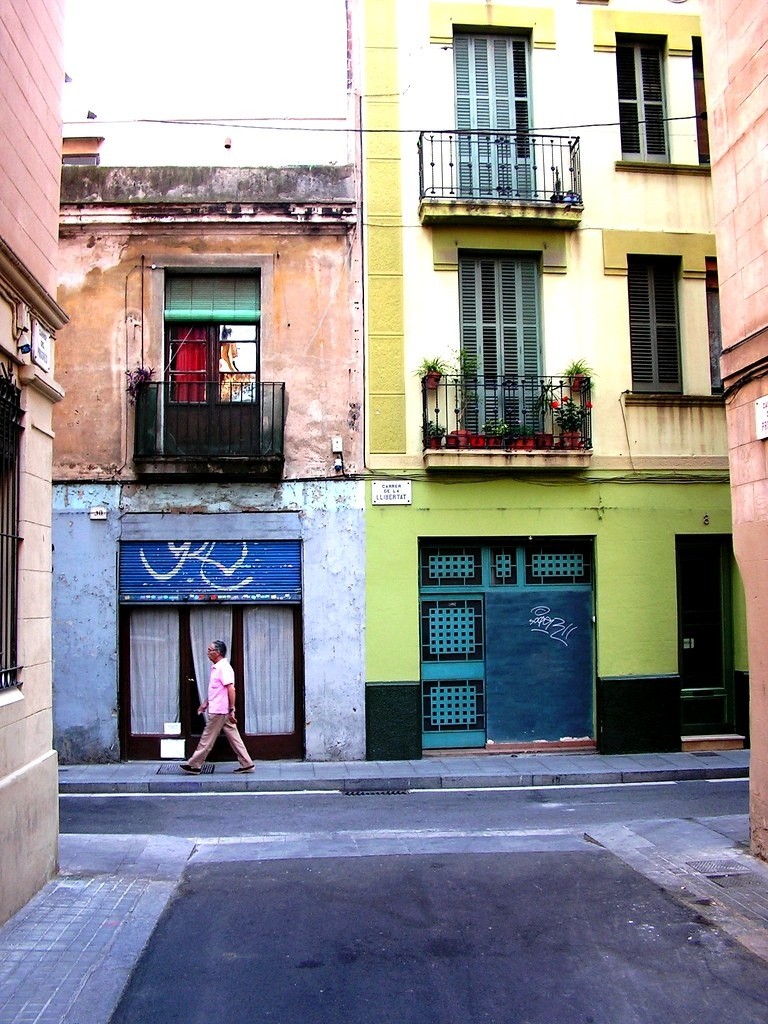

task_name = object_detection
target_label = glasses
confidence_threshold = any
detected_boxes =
[208,648,219,652]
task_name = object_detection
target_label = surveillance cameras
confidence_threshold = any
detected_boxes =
[225,138,232,149]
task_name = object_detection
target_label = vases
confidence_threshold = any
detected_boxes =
[469,434,485,448]
[559,433,580,450]
[445,434,458,449]
[536,433,553,450]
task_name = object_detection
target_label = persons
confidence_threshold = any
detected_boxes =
[179,640,255,774]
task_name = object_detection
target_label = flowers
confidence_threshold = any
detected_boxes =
[550,396,592,434]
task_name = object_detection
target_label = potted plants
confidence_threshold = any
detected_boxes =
[452,348,479,449]
[420,421,446,449]
[481,418,508,448]
[556,359,600,393]
[416,358,451,390]
[508,423,535,448]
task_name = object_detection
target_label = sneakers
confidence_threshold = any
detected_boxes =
[179,764,202,775]
[234,765,256,773]
